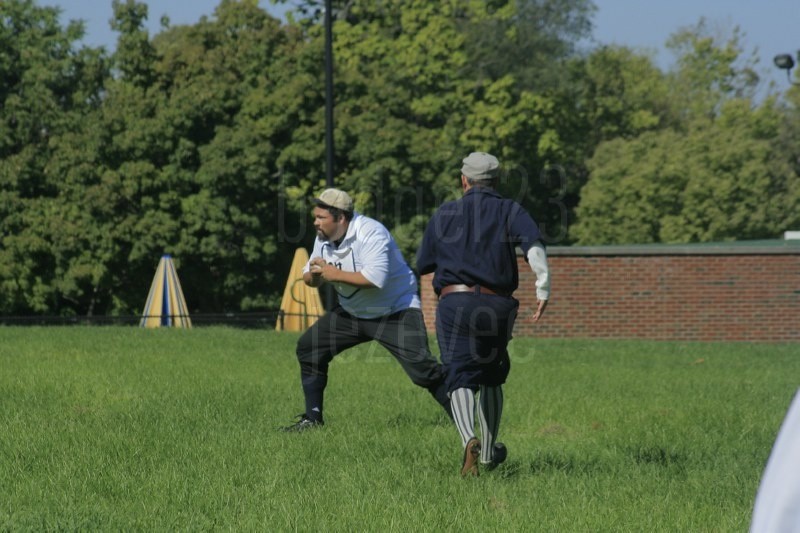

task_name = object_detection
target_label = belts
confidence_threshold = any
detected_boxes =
[440,284,512,299]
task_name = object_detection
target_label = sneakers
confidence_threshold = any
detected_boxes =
[281,414,324,434]
[461,437,480,479]
[481,442,507,471]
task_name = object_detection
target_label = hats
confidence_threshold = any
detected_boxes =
[318,189,353,213]
[461,151,499,180]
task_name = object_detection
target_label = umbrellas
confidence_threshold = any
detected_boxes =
[275,247,325,333]
[138,255,191,328]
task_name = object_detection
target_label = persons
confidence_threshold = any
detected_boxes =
[416,152,551,478]
[277,188,442,433]
